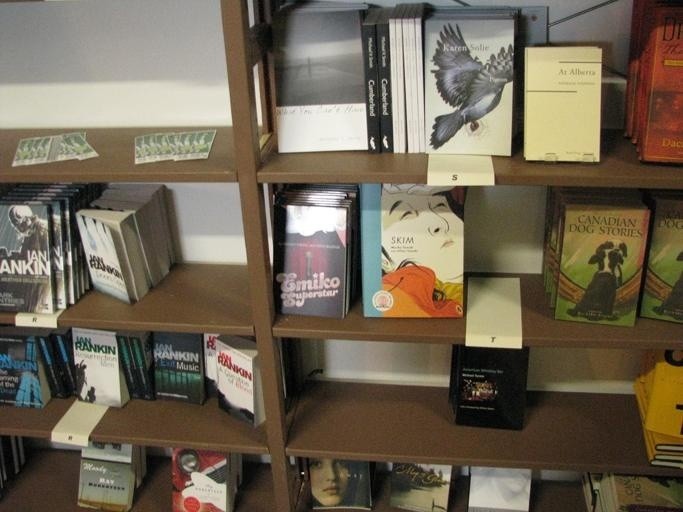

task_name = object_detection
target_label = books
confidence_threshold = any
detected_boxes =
[640,188,683,325]
[449,343,529,431]
[77,442,145,512]
[525,46,602,166]
[150,331,205,406]
[469,466,532,512]
[77,184,174,305]
[0,327,77,410]
[272,0,521,157]
[172,447,241,512]
[116,328,141,401]
[129,330,155,402]
[218,335,265,428]
[581,470,683,512]
[307,455,371,511]
[204,332,223,398]
[390,462,452,511]
[0,435,26,494]
[274,185,360,320]
[542,186,650,327]
[359,183,463,318]
[0,182,102,314]
[633,350,683,469]
[72,326,129,409]
[625,0,683,164]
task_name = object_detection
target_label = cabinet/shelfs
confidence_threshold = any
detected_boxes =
[0,0,681,507]
